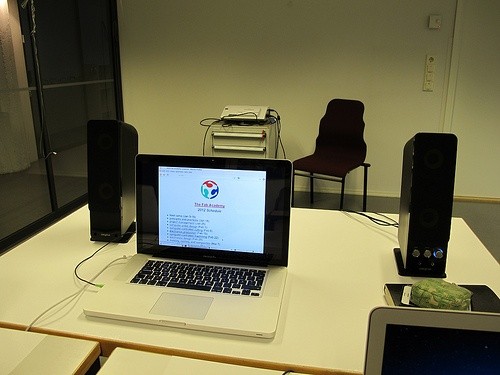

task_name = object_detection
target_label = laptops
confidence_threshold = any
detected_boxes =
[363,306,500,375]
[81,154,296,339]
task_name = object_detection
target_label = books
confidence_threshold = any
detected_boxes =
[384,284,500,313]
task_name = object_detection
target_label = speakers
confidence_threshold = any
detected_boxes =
[87,119,139,239]
[398,132,459,276]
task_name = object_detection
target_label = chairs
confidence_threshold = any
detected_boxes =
[290,98,371,213]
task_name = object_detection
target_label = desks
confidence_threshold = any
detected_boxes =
[0,203,500,375]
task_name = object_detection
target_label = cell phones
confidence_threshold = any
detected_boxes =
[401,286,471,311]
